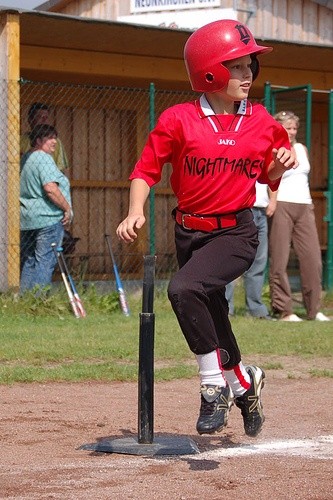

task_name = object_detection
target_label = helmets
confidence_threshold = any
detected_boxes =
[185,19,273,94]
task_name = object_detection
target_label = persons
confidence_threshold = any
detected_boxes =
[18,123,73,303]
[115,19,295,439]
[268,110,331,323]
[18,103,69,173]
[225,180,279,323]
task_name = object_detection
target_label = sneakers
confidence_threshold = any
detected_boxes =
[196,380,234,435]
[233,365,265,437]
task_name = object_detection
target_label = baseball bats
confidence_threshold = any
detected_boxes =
[105,234,130,318]
[56,247,85,317]
[51,242,80,319]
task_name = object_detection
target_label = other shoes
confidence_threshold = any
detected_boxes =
[313,311,331,322]
[280,313,303,322]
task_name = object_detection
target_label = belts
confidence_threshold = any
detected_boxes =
[174,209,254,233]
